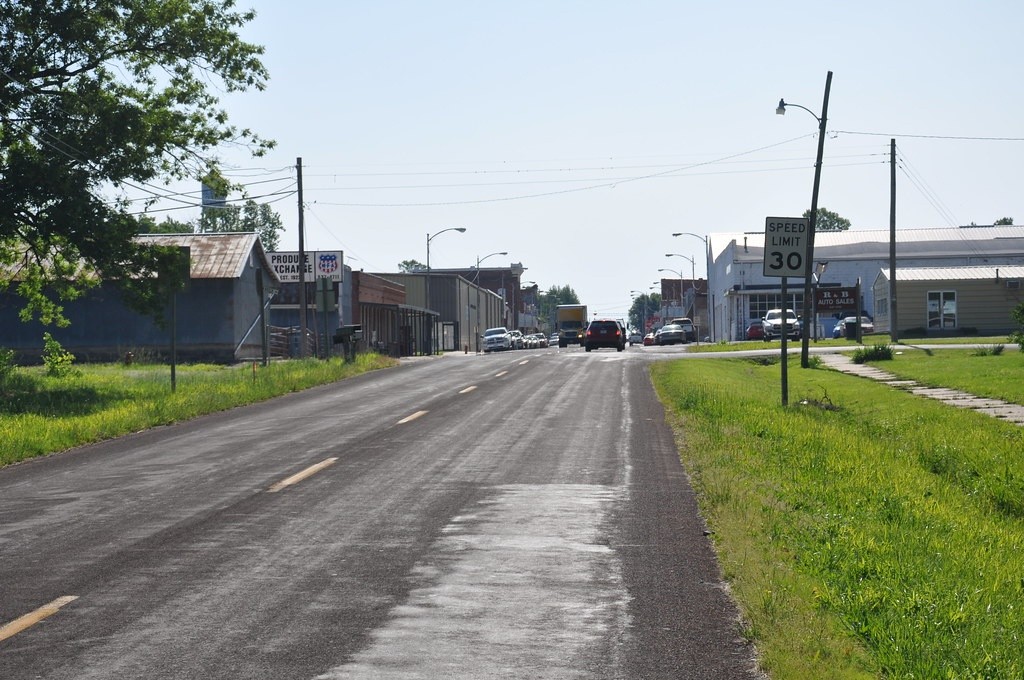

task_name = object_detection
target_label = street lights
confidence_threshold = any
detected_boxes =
[477,252,507,354]
[773,71,833,369]
[631,282,666,334]
[516,281,536,336]
[658,268,684,318]
[665,254,695,344]
[672,232,710,346]
[426,227,467,357]
[539,290,562,333]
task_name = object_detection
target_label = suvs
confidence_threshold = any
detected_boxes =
[628,332,643,347]
[762,309,802,342]
[671,318,696,342]
[586,319,626,352]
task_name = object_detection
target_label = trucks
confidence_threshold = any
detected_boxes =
[557,304,587,346]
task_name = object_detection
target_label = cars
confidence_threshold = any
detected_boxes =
[644,334,654,345]
[833,317,874,339]
[654,325,686,346]
[550,333,559,346]
[745,322,763,340]
[480,327,548,351]
